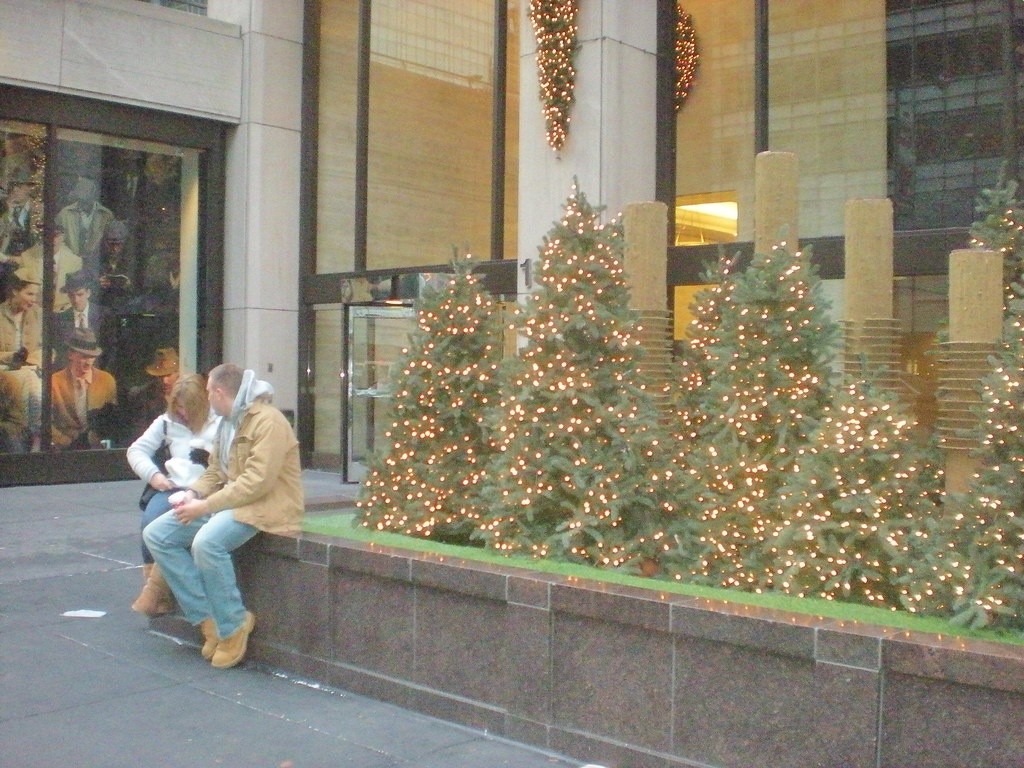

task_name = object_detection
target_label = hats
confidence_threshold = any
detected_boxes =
[7,163,37,185]
[73,176,97,203]
[63,328,103,356]
[13,267,43,285]
[145,347,179,376]
[104,221,129,244]
[59,270,100,293]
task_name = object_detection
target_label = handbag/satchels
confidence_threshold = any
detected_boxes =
[139,420,173,512]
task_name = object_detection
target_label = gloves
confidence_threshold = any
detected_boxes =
[9,346,28,371]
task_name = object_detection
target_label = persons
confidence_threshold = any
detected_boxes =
[126,371,222,618]
[0,134,182,452]
[142,362,305,668]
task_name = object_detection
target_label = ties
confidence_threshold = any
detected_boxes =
[128,179,136,196]
[78,379,86,400]
[11,209,20,225]
[78,313,84,327]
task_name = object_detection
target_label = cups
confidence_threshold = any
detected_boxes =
[167,491,190,524]
[100,440,110,449]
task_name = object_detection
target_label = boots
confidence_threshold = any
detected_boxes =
[131,562,173,617]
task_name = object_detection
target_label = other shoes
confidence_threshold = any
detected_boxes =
[200,618,219,661]
[211,611,255,669]
